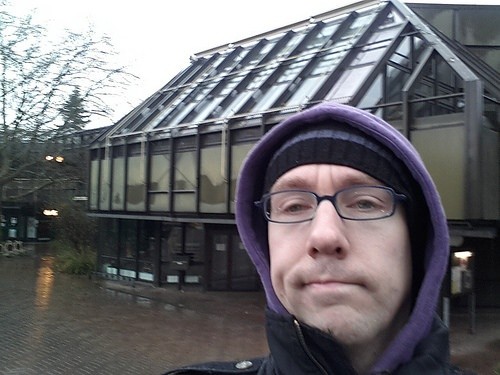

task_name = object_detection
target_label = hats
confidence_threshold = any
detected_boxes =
[260,122,421,223]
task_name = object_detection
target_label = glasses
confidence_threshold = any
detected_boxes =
[252,183,411,235]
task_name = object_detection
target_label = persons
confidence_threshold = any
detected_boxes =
[157,102,473,374]
